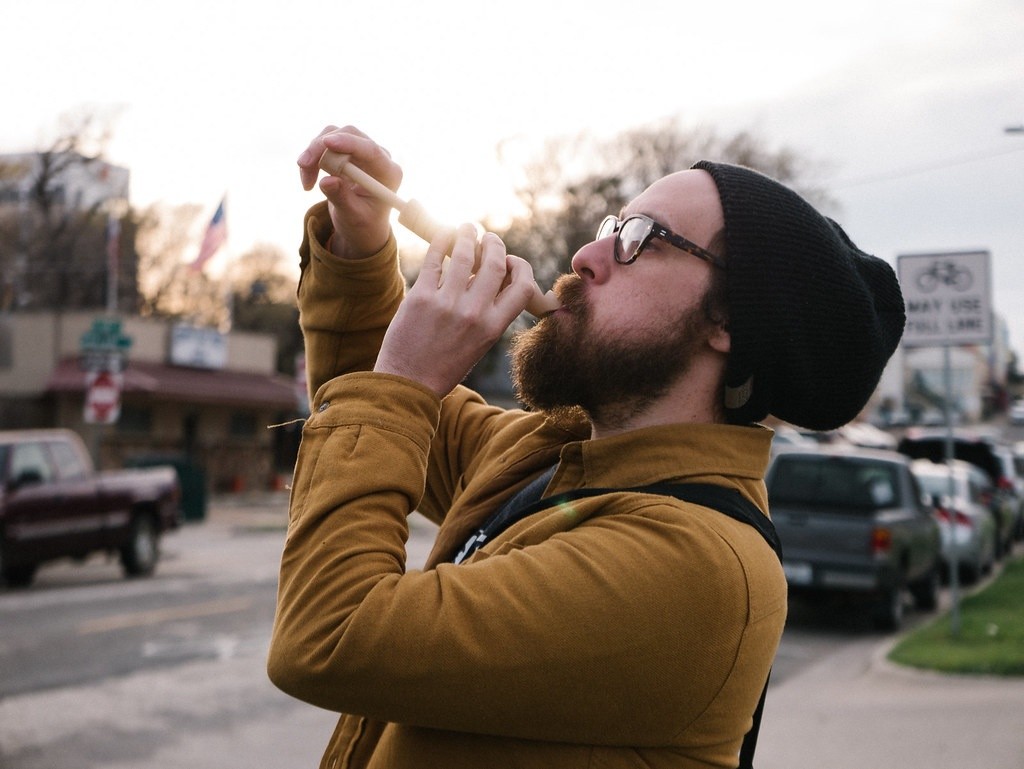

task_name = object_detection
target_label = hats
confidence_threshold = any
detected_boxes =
[690,159,907,431]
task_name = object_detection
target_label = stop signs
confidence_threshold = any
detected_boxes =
[88,367,121,420]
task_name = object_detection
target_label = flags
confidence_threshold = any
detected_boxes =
[192,204,228,270]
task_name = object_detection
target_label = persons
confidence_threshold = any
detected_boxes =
[268,125,907,769]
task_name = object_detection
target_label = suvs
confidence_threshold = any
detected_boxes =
[762,422,1024,634]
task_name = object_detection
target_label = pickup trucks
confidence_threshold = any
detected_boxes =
[1,429,186,591]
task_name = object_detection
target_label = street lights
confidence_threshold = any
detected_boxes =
[107,196,129,383]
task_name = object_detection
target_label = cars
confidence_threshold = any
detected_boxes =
[1007,399,1023,419]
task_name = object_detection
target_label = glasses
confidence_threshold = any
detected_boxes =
[596,212,723,282]
